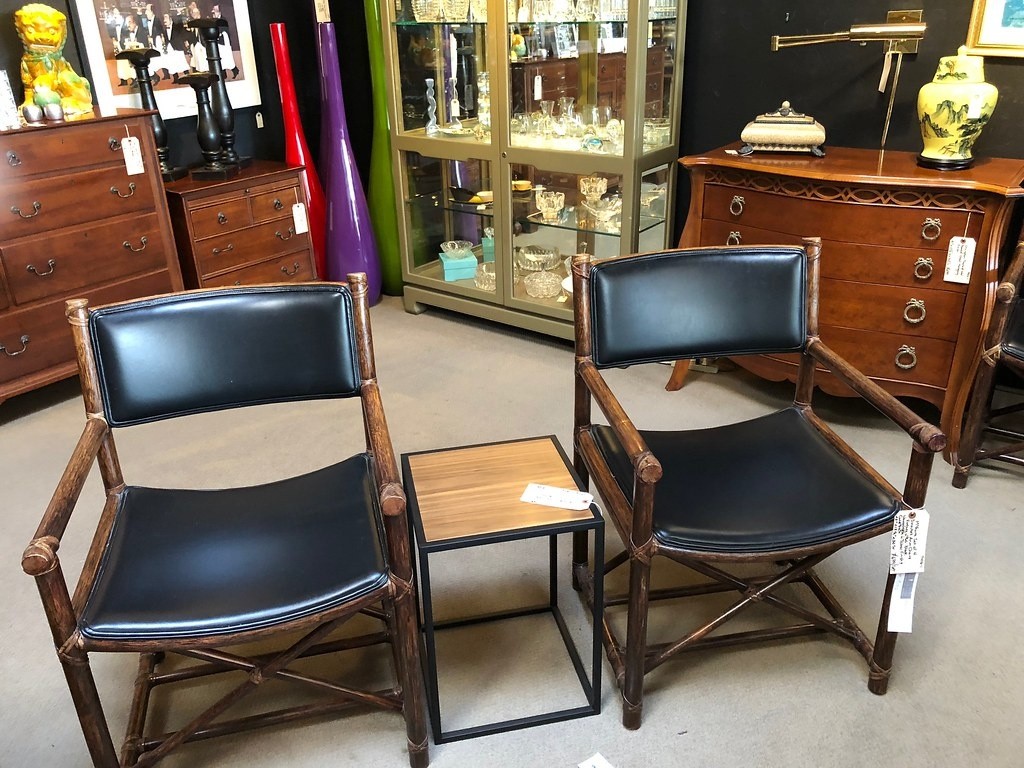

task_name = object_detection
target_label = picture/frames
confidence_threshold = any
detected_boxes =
[66,0,264,128]
[966,0,1024,59]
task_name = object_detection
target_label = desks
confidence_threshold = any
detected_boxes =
[401,435,604,745]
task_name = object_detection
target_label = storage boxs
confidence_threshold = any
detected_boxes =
[481,237,494,262]
[438,252,479,281]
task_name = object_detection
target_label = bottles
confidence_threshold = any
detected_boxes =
[918,45,998,170]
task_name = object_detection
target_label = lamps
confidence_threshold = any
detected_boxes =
[771,9,926,146]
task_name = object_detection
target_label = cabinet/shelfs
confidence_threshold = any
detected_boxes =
[666,141,1024,464]
[164,159,319,289]
[508,45,667,235]
[380,0,688,341]
[0,106,185,405]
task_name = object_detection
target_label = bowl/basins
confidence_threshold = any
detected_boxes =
[449,186,478,201]
[440,241,473,258]
[476,190,493,202]
[512,180,531,191]
[561,277,573,306]
[581,200,621,222]
[563,254,599,276]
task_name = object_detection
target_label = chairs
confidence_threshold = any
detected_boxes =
[952,223,1024,488]
[21,272,432,767]
[571,236,948,730]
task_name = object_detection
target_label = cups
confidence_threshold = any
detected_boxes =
[411,0,678,23]
[510,96,613,136]
